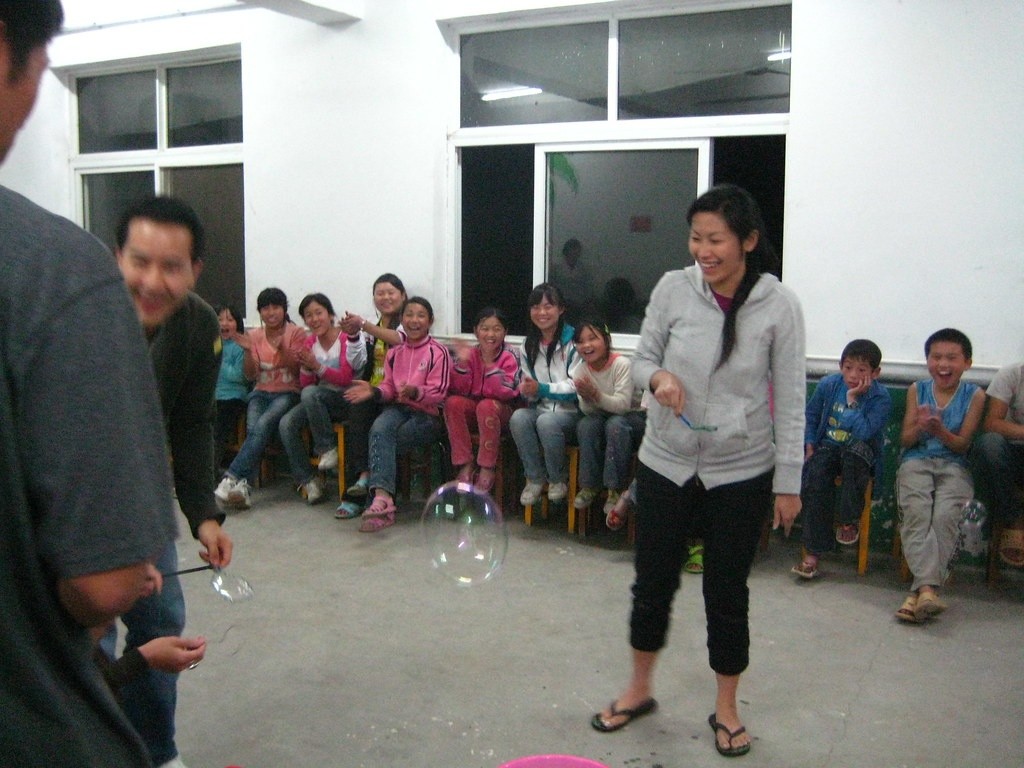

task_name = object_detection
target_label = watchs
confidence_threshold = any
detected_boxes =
[848,400,858,409]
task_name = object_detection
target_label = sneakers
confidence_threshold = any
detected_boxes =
[520,479,546,506]
[573,484,600,510]
[228,479,252,509]
[305,477,324,503]
[603,488,622,515]
[214,477,234,501]
[318,446,340,469]
[548,481,567,499]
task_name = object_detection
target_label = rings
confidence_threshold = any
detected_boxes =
[189,663,198,670]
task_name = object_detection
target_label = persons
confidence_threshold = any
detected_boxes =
[590,185,806,758]
[99,196,234,768]
[443,307,527,495]
[343,296,454,533]
[977,364,1024,567]
[279,293,354,503]
[509,283,582,507]
[335,273,409,519]
[791,339,892,579]
[0,0,208,768]
[895,328,986,624]
[213,288,307,510]
[213,300,249,444]
[574,316,650,532]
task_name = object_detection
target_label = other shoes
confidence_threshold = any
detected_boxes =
[473,472,497,495]
[455,462,477,494]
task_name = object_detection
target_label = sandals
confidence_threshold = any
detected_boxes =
[605,491,634,531]
[682,544,704,574]
[359,517,396,532]
[362,496,396,518]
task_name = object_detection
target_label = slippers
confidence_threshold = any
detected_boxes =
[709,712,752,754]
[833,520,861,544]
[334,500,364,517]
[791,561,819,578]
[999,527,1024,567]
[347,477,369,496]
[914,593,948,618]
[591,694,658,732]
[894,596,918,623]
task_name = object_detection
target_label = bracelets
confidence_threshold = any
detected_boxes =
[360,320,365,331]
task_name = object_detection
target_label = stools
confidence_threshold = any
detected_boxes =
[232,413,1000,582]
